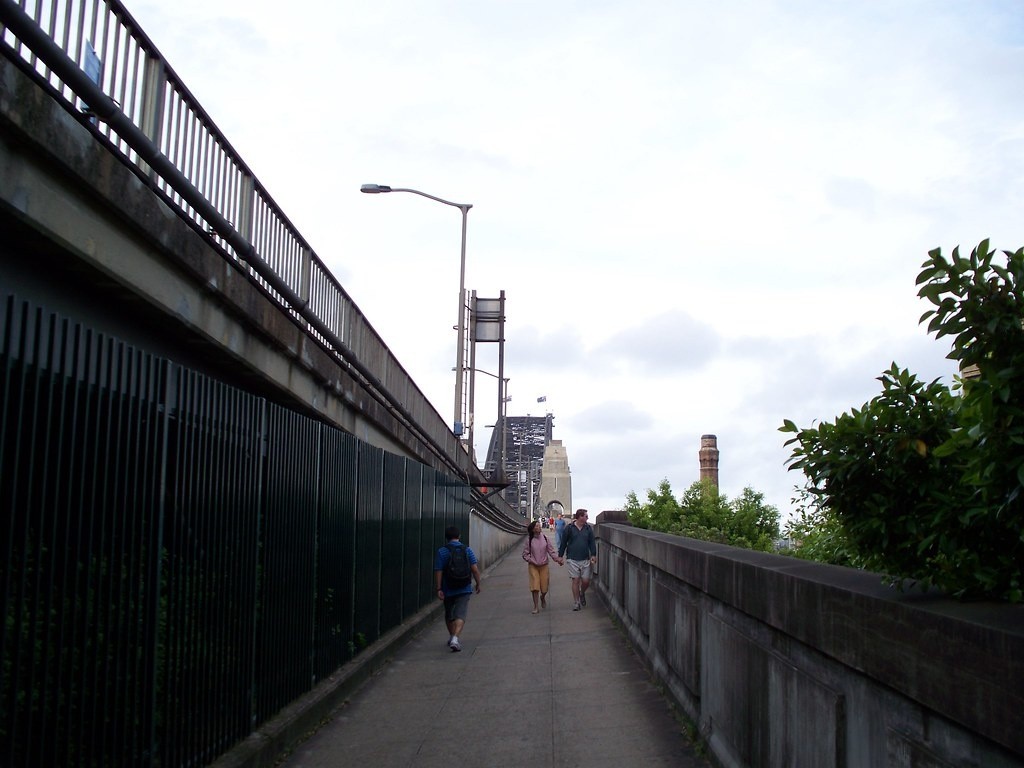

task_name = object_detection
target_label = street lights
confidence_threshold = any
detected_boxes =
[483,425,522,514]
[451,367,510,500]
[360,185,472,442]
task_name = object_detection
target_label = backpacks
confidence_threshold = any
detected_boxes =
[444,543,471,590]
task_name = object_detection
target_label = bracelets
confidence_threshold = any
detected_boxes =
[437,589,442,591]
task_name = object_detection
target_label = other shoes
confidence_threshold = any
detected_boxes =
[580,594,586,606]
[448,640,462,651]
[532,608,539,614]
[540,596,546,608]
[573,602,581,610]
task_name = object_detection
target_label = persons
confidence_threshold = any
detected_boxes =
[434,526,480,652]
[522,522,563,614]
[549,516,554,531]
[555,513,565,555]
[558,510,598,612]
